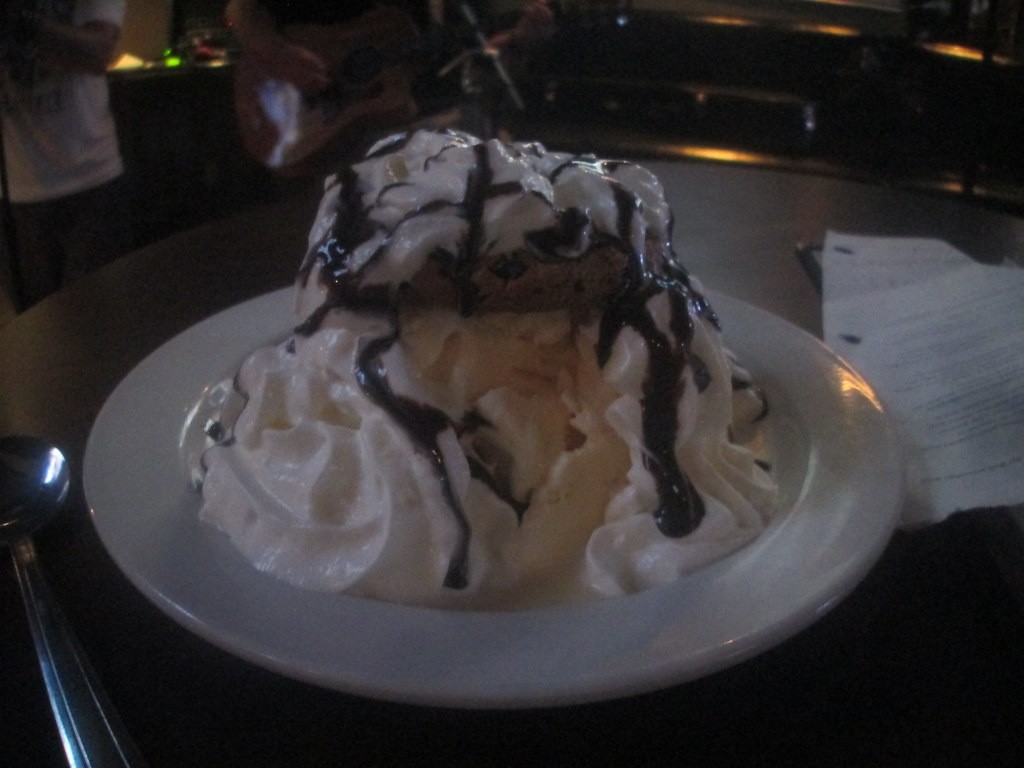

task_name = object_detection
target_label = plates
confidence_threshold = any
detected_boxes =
[84,284,905,708]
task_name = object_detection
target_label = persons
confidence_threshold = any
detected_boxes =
[0,0,135,321]
[220,0,556,189]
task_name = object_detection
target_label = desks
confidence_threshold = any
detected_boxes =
[98,63,251,238]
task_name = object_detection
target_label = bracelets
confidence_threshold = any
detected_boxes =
[14,9,43,40]
[505,28,518,48]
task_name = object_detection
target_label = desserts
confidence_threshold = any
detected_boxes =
[198,125,786,611]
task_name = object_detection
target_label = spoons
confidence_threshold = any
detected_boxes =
[0,434,145,768]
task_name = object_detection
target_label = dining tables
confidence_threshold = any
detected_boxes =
[0,165,1024,768]
